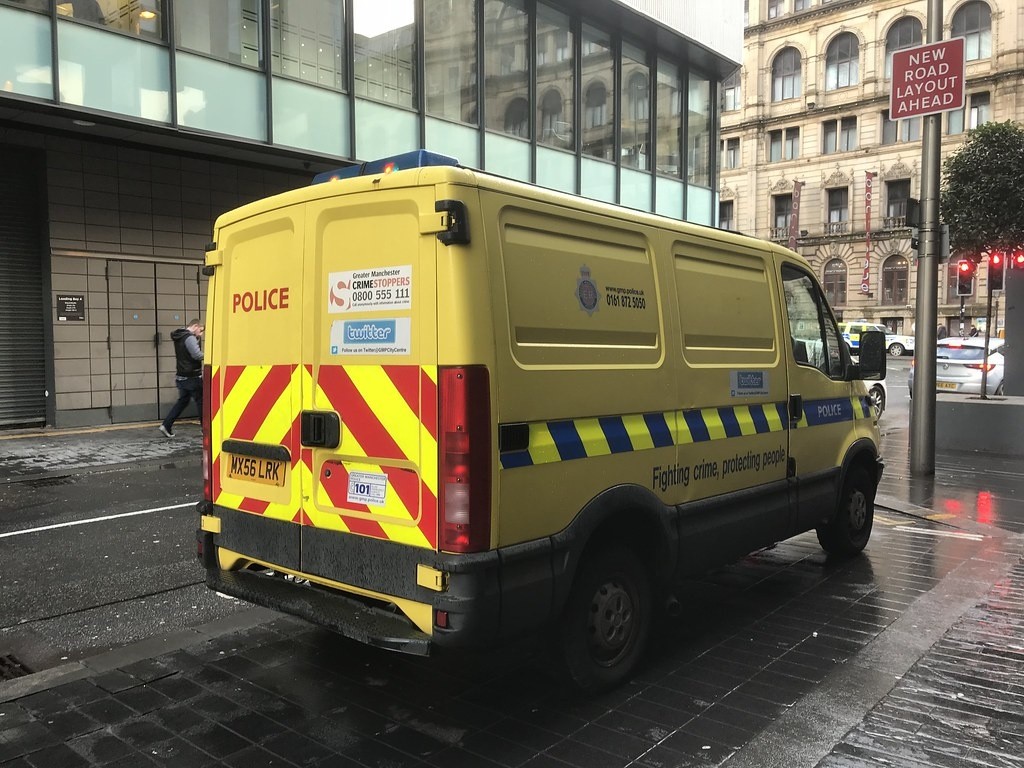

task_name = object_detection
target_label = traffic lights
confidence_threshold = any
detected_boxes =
[1013,251,1024,269]
[957,259,973,296]
[987,252,1004,291]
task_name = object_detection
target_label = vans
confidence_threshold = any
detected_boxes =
[193,148,887,707]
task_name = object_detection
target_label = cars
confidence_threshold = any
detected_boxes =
[908,336,1004,398]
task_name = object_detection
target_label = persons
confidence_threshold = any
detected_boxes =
[969,325,978,337]
[937,323,946,340]
[158,319,204,438]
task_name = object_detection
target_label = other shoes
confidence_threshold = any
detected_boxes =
[159,424,176,437]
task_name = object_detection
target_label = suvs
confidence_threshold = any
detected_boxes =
[835,322,917,357]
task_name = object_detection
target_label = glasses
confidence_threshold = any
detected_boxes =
[199,328,204,333]
